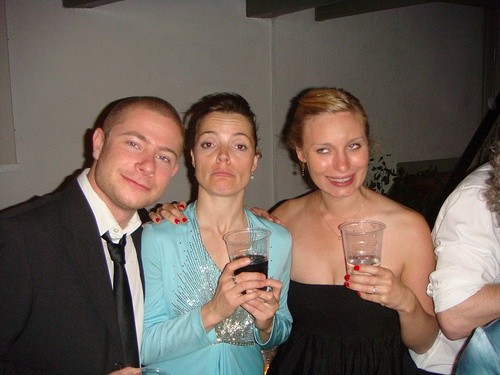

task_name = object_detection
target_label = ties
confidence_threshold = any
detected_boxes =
[101,234,140,368]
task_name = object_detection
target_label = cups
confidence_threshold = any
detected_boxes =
[338,220,386,276]
[141,366,169,375]
[222,227,272,296]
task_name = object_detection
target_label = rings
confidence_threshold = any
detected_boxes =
[232,276,237,285]
[374,286,378,294]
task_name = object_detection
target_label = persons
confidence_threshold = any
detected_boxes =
[149,87,439,375]
[0,96,286,375]
[399,130,500,375]
[140,92,294,375]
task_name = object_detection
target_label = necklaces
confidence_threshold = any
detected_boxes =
[319,187,368,241]
